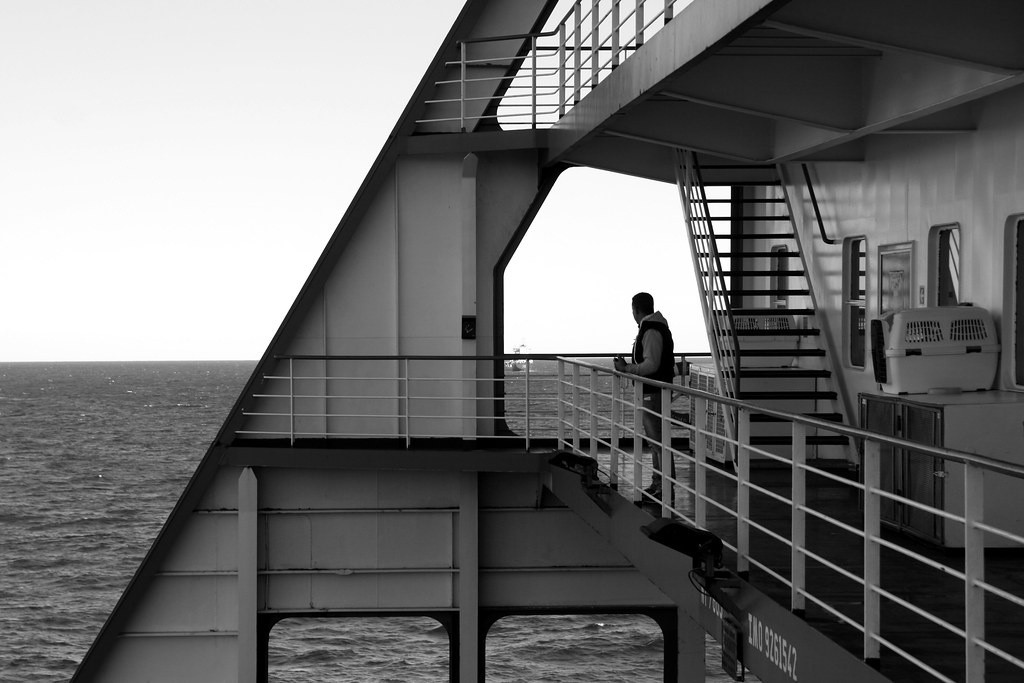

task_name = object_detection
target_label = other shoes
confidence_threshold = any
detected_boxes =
[643,485,675,501]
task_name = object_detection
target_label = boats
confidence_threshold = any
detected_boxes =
[510,347,532,370]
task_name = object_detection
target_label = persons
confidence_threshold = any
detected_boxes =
[613,292,676,504]
